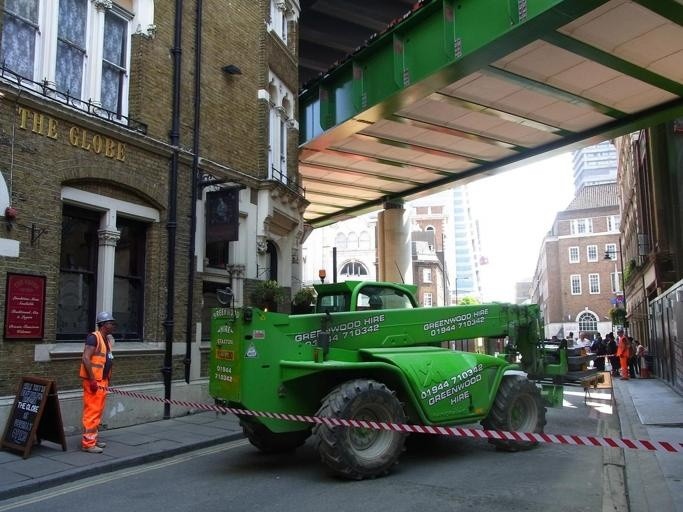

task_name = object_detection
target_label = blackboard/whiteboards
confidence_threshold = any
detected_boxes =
[0,375,53,452]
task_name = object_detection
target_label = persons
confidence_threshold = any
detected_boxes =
[79,312,116,453]
[551,327,647,380]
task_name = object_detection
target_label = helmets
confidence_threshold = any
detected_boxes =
[96,312,116,324]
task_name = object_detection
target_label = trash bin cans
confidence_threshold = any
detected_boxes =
[641,355,657,379]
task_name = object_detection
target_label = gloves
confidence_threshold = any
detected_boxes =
[90,379,98,392]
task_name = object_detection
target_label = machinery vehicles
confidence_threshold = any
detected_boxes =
[208,280,569,482]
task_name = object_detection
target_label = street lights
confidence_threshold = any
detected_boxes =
[604,237,631,336]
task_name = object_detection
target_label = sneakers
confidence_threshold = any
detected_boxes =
[81,441,106,453]
